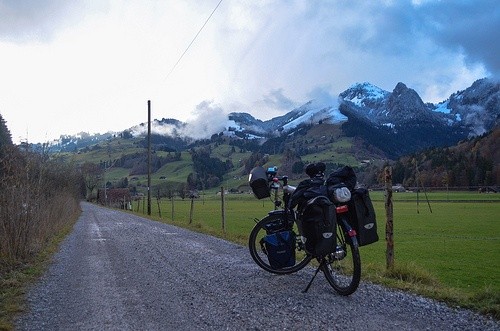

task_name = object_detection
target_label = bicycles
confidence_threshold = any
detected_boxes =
[249,159,379,296]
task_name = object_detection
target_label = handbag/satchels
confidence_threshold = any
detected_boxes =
[300,194,337,257]
[346,186,381,247]
[263,231,298,269]
[248,164,269,199]
